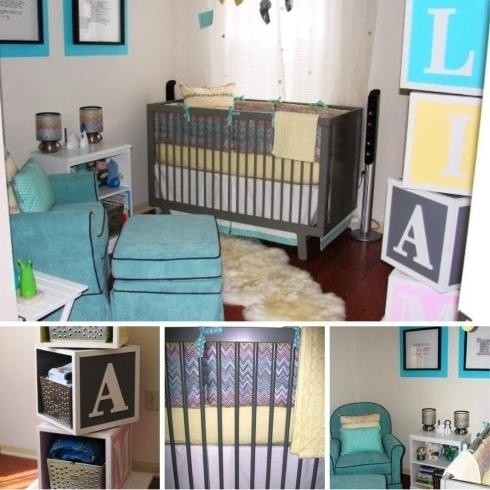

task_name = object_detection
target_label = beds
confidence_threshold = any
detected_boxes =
[145,96,364,261]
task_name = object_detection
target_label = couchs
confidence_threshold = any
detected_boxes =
[109,213,227,321]
[4,153,110,320]
[331,400,407,489]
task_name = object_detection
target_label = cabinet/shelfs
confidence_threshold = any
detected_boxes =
[30,141,135,256]
[408,426,471,488]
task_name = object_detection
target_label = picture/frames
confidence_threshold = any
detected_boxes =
[403,326,441,371]
[465,326,489,372]
[0,0,45,45]
[71,0,127,46]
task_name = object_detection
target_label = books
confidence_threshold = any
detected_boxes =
[415,466,434,489]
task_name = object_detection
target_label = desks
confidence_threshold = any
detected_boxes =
[16,268,89,324]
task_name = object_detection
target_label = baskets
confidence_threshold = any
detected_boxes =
[46,458,105,488]
[39,375,72,429]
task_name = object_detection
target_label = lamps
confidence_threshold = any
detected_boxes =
[421,406,437,432]
[35,110,63,154]
[78,106,105,145]
[453,410,471,435]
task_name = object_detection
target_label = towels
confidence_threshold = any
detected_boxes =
[270,110,320,164]
[288,326,325,459]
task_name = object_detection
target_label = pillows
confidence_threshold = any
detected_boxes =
[12,157,56,213]
[338,425,386,456]
[179,82,235,110]
[341,412,381,428]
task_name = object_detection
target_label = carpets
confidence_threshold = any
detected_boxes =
[219,234,349,322]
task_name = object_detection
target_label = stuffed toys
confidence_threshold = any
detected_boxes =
[86,158,122,189]
[426,442,459,463]
